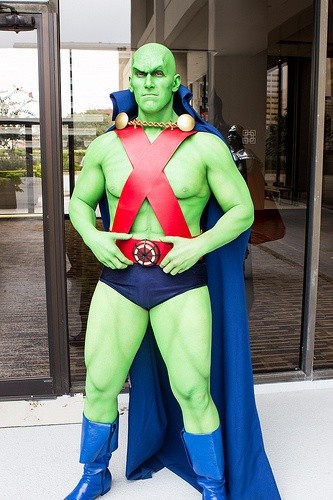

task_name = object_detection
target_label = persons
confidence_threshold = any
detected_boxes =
[64,43,282,500]
[65,96,256,347]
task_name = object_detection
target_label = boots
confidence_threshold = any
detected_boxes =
[64,411,119,500]
[180,424,227,500]
